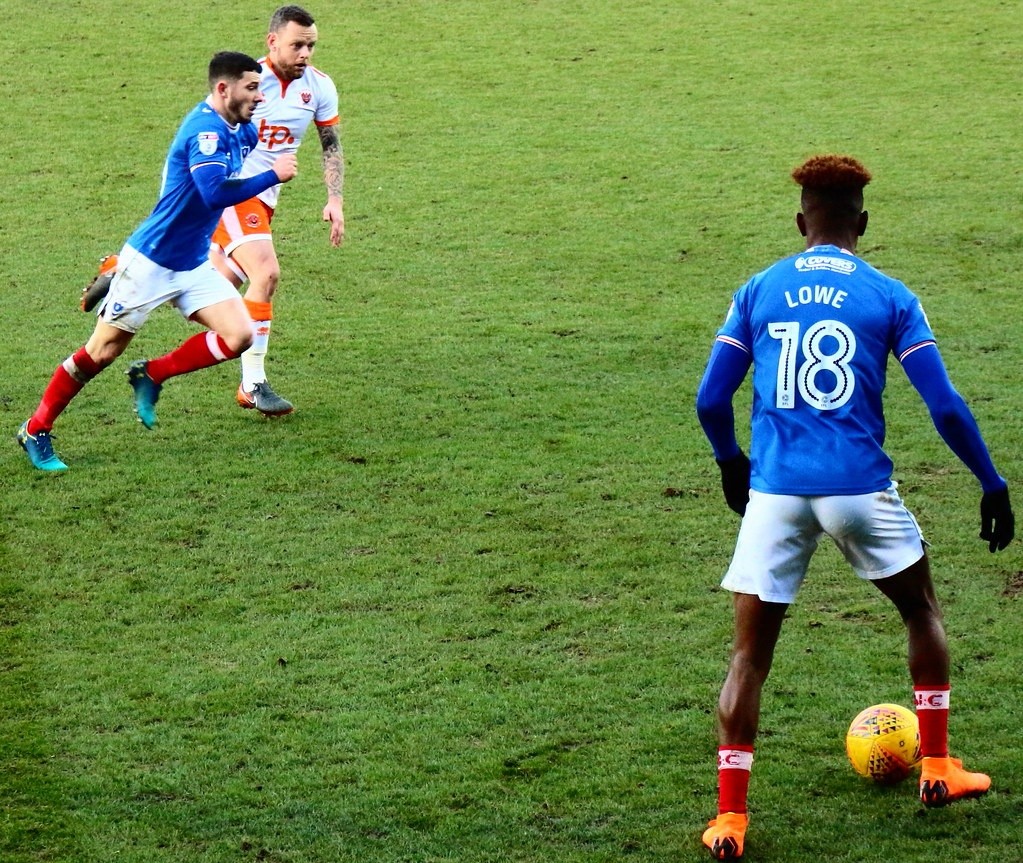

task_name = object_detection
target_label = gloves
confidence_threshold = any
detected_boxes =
[715,448,750,518]
[979,484,1015,553]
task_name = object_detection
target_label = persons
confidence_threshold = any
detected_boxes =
[696,155,1017,858]
[78,5,346,417]
[13,52,299,472]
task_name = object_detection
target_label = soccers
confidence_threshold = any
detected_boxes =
[847,701,922,786]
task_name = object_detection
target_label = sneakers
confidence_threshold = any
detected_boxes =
[79,254,118,312]
[123,359,163,430]
[236,378,296,417]
[16,417,69,471]
[701,812,750,861]
[919,755,992,809]
